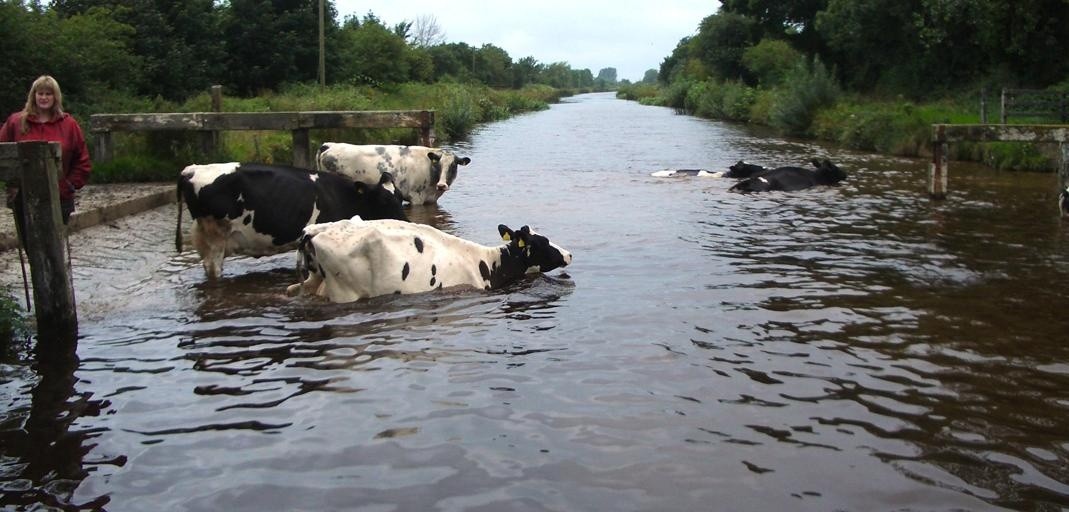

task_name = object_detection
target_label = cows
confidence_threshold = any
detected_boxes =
[176,161,412,283]
[727,158,848,192]
[287,219,572,303]
[651,161,769,178]
[315,142,470,205]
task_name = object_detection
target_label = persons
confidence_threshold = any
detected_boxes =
[0,76,94,225]
